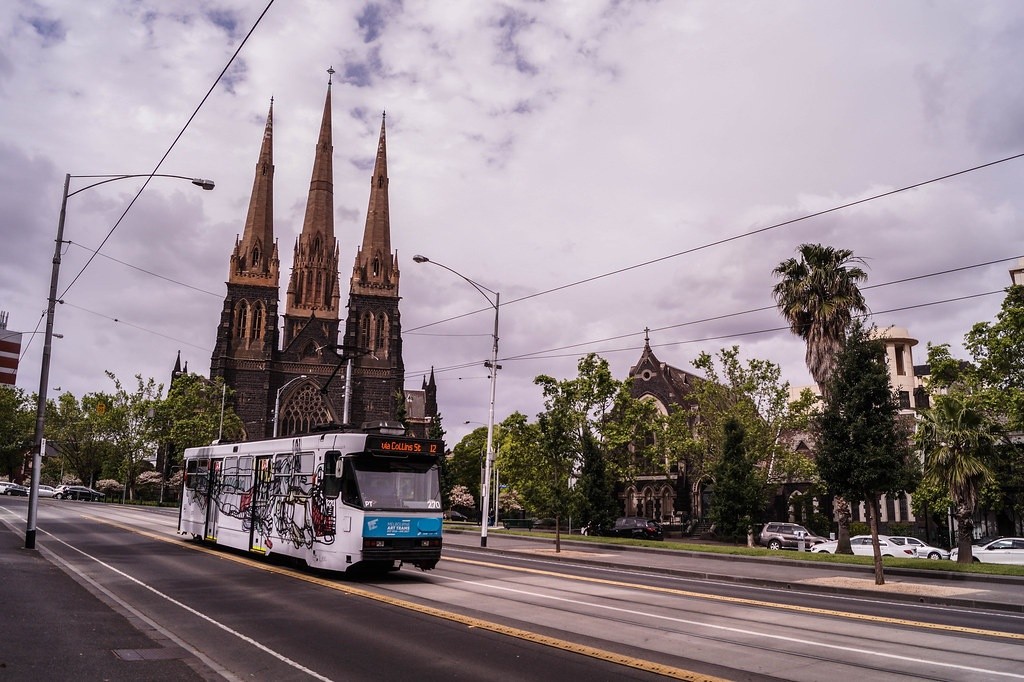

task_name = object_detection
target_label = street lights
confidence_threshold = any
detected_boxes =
[413,255,500,547]
[24,173,215,549]
[176,370,226,442]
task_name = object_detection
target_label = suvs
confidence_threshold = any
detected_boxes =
[53,485,68,500]
[758,521,826,551]
[64,485,105,502]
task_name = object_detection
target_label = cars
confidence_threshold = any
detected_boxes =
[4,485,28,497]
[810,534,919,559]
[27,484,56,499]
[584,516,664,541]
[948,538,1024,566]
[891,537,951,561]
[0,482,17,494]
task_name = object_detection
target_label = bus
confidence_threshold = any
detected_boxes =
[177,420,445,582]
